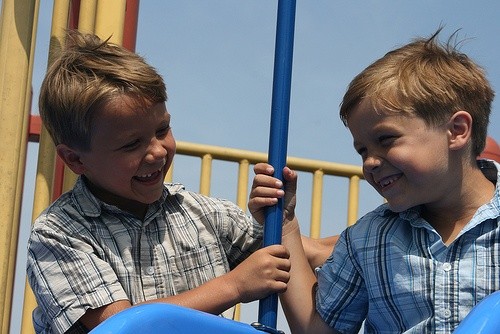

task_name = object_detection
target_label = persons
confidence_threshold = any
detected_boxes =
[246,25,499,333]
[20,29,346,333]
